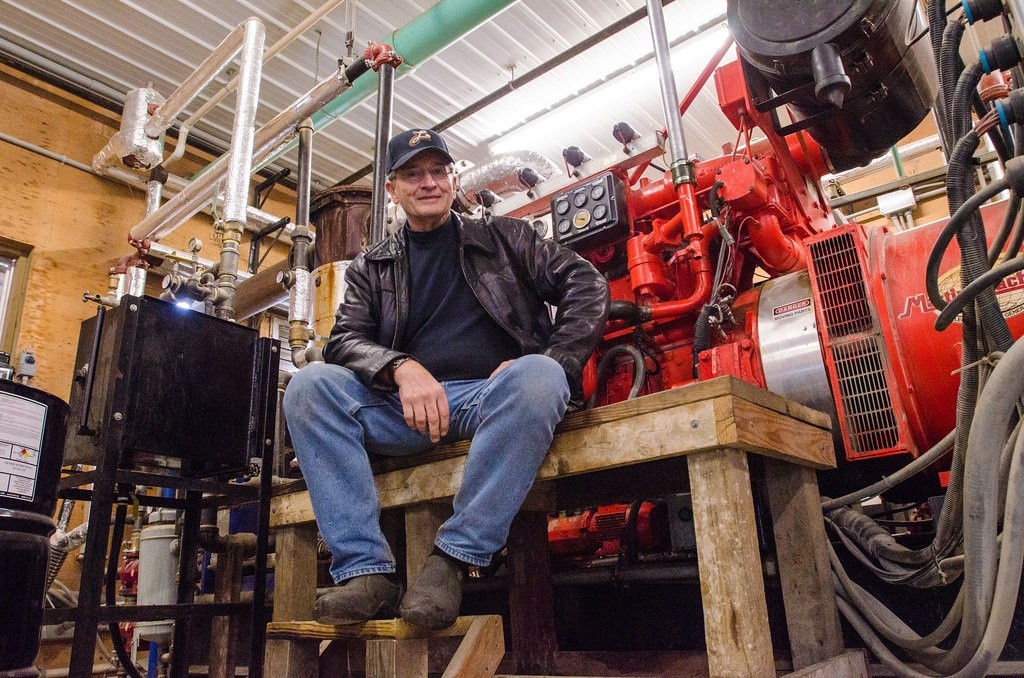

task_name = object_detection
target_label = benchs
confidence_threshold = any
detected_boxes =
[262,373,870,677]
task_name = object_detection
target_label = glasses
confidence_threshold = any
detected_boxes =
[396,162,453,182]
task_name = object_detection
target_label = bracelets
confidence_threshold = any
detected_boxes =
[392,357,410,368]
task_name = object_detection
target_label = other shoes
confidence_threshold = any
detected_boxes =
[311,572,406,626]
[400,552,465,631]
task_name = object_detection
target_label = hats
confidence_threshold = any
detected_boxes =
[383,128,458,176]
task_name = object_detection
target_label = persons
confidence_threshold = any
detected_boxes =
[281,126,611,627]
